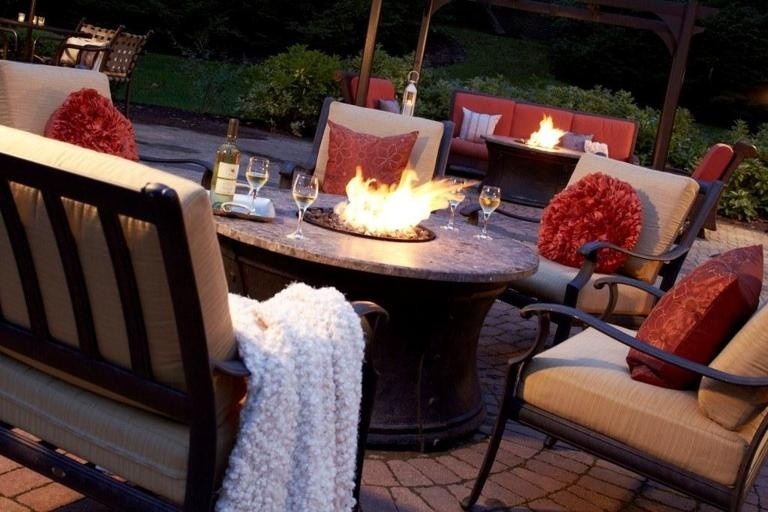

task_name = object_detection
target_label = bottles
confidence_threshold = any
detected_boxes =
[210,119,240,209]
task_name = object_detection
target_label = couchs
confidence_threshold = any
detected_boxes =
[687,140,760,239]
[338,71,394,111]
[442,89,641,175]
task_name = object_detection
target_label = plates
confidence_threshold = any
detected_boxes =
[205,189,277,223]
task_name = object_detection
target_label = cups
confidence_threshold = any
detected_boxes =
[286,172,318,243]
[440,178,466,235]
[473,186,502,240]
[244,157,269,214]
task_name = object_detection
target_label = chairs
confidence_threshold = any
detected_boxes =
[29,16,117,66]
[56,24,154,120]
[0,59,112,138]
[496,151,725,348]
[458,276,768,512]
[301,95,457,216]
[0,125,389,512]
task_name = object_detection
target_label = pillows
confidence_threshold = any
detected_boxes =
[561,129,594,152]
[626,244,764,388]
[43,87,140,164]
[320,119,419,196]
[460,106,502,145]
[538,170,644,275]
[583,138,609,160]
